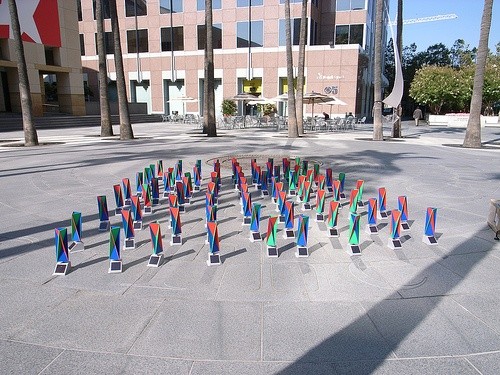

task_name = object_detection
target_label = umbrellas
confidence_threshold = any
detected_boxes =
[231,91,347,118]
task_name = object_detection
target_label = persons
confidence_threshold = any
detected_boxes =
[413,106,422,126]
[346,112,354,119]
[323,112,329,120]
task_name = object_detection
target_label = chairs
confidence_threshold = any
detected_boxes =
[159,113,366,132]
